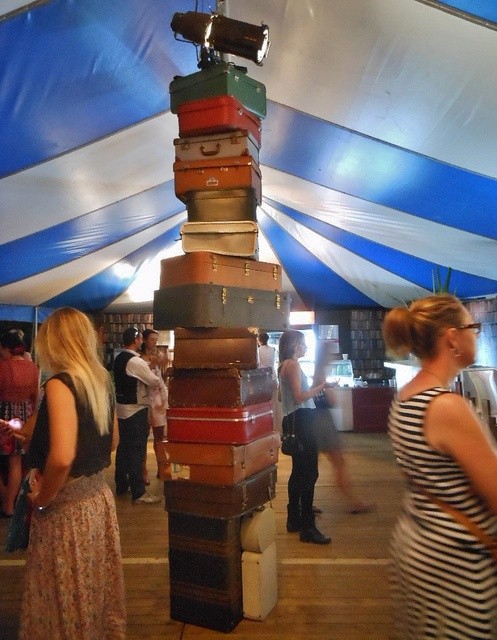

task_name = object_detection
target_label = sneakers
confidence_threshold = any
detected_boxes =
[136,491,161,503]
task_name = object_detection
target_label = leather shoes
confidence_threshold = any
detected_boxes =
[300,527,330,544]
[287,520,316,532]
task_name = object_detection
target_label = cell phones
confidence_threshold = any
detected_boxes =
[1,418,26,442]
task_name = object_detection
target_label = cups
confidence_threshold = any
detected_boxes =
[387,379,393,387]
[341,354,348,361]
[393,379,396,388]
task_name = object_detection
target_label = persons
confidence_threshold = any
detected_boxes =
[254,329,277,369]
[1,328,40,519]
[382,292,497,640]
[311,341,378,514]
[113,326,170,505]
[17,306,126,640]
[276,329,340,545]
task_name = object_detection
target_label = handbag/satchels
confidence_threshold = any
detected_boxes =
[8,472,30,553]
[405,474,496,561]
[281,360,304,455]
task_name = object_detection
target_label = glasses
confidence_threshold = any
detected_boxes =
[134,326,139,337]
[455,323,480,335]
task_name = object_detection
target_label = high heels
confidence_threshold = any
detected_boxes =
[144,478,150,486]
